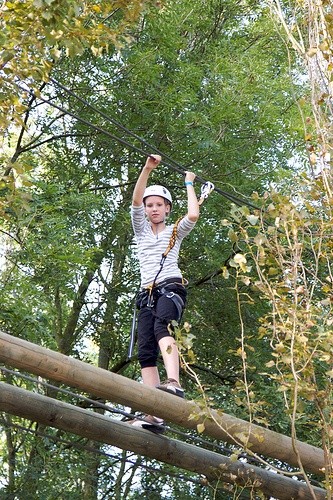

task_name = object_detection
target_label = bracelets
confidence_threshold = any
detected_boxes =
[185,181,194,186]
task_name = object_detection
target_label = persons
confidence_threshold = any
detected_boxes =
[129,153,199,434]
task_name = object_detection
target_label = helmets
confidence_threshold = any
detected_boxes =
[142,184,173,241]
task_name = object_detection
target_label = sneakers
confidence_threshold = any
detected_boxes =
[155,378,186,398]
[122,412,165,432]
